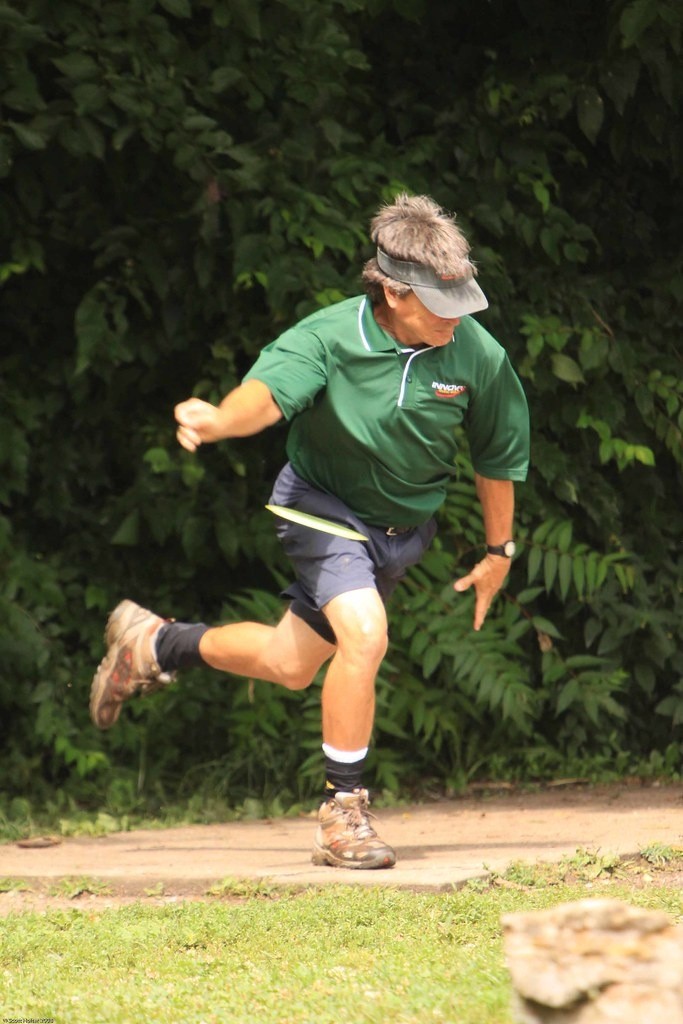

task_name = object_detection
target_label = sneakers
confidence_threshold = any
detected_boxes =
[87,599,179,730]
[310,788,396,870]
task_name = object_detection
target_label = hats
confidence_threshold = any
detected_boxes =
[376,246,490,319]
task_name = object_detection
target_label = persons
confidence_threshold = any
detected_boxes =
[89,192,530,871]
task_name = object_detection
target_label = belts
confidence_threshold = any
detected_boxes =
[375,525,410,536]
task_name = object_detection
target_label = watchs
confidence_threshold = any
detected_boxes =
[487,540,515,558]
